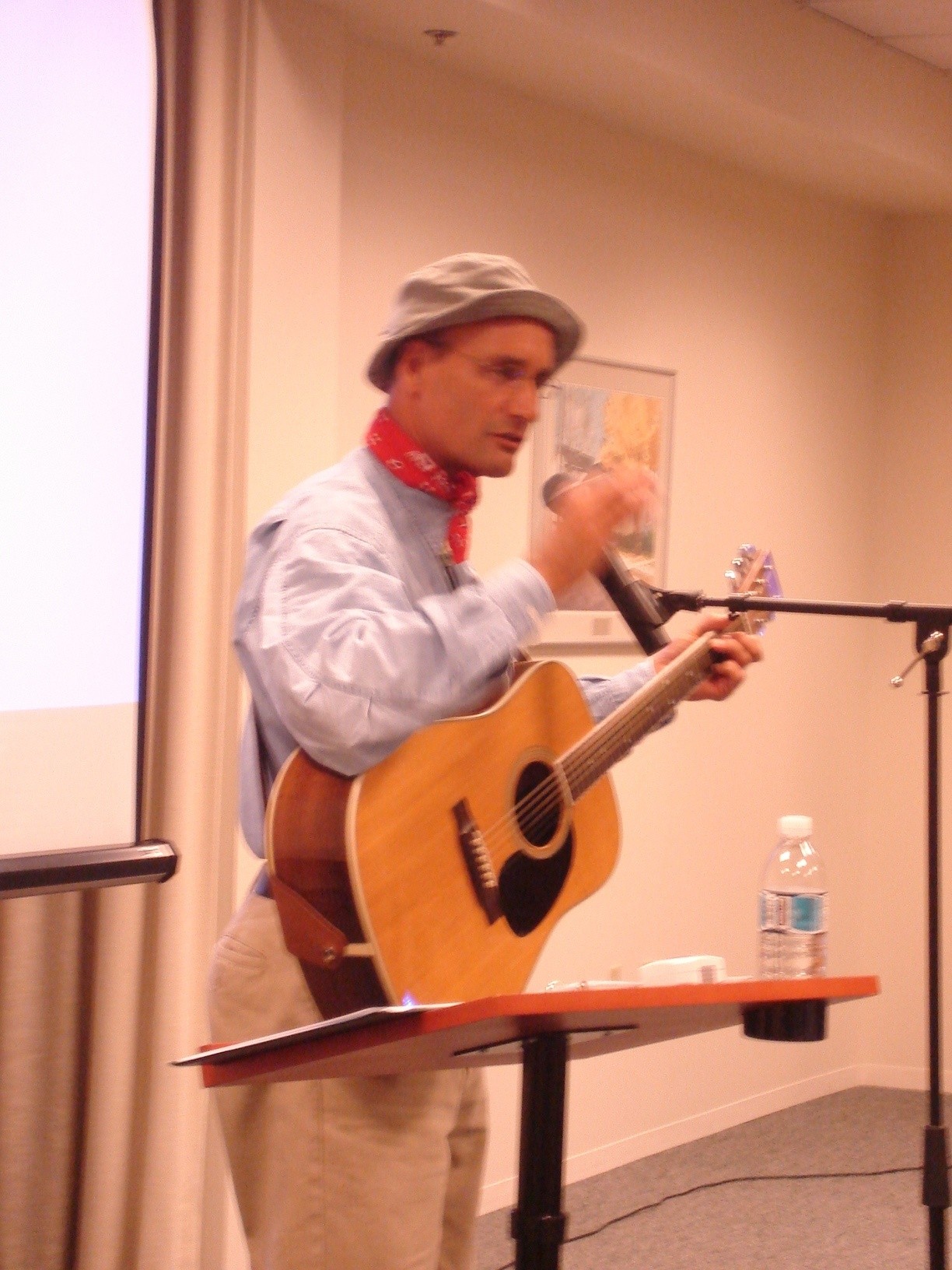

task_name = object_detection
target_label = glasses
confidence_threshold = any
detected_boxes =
[422,338,563,401]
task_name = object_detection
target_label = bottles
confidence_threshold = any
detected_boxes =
[756,815,829,981]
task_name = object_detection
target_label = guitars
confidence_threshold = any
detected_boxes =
[262,539,784,1020]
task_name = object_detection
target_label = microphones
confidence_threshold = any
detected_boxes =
[542,472,672,657]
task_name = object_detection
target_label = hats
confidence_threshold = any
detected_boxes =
[368,251,588,388]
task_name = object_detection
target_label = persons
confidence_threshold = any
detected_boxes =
[203,252,764,1270]
